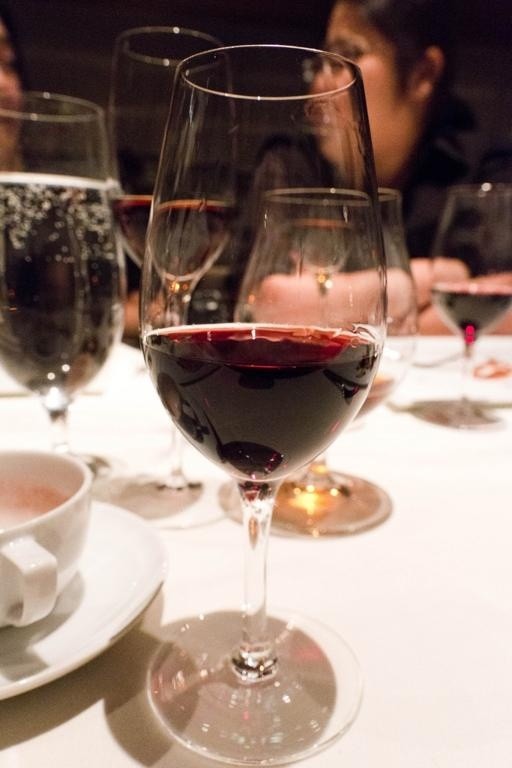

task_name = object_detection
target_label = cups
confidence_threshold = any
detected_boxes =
[1,447,98,634]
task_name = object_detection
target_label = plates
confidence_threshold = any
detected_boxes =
[1,499,169,702]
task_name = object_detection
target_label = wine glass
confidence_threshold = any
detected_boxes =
[140,36,394,767]
[1,92,126,482]
[213,184,424,544]
[428,181,512,436]
[106,24,242,324]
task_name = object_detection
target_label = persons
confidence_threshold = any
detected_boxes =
[0,18,29,167]
[231,0,512,338]
